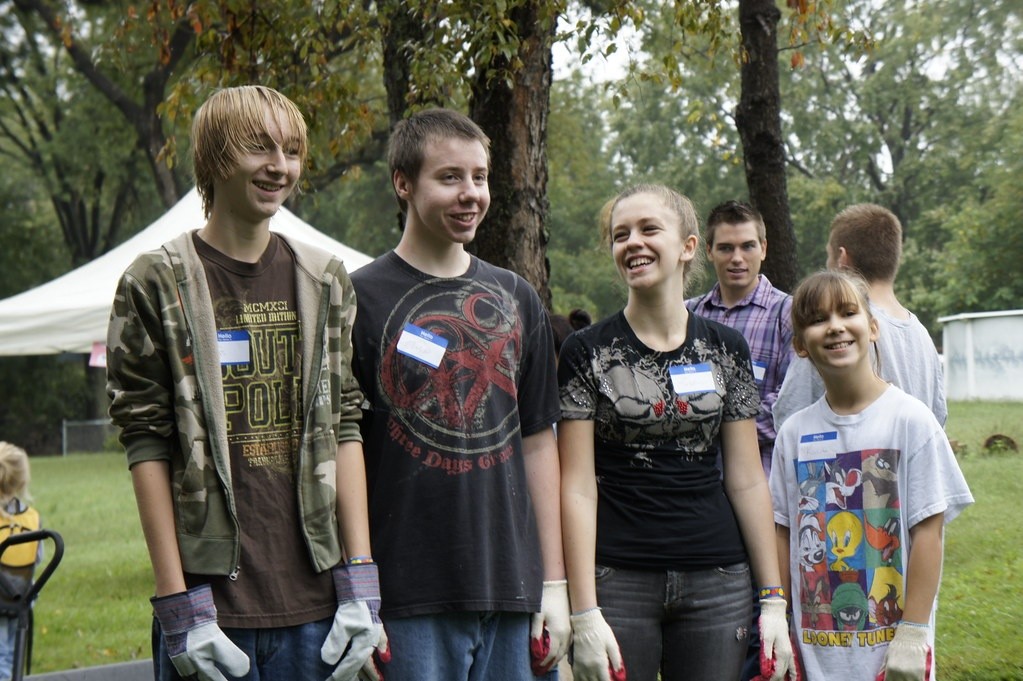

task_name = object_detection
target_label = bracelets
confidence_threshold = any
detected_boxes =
[350,556,373,564]
[759,587,785,600]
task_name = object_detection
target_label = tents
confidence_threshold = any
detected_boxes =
[0,187,375,355]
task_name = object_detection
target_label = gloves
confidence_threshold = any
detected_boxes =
[358,627,391,681]
[150,584,250,681]
[530,579,573,676]
[321,562,382,681]
[570,607,626,681]
[750,599,802,681]
[876,620,932,681]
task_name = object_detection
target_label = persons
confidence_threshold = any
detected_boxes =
[103,85,383,681]
[684,199,796,481]
[769,269,974,681]
[558,185,796,681]
[348,109,573,681]
[0,439,43,681]
[771,203,948,437]
